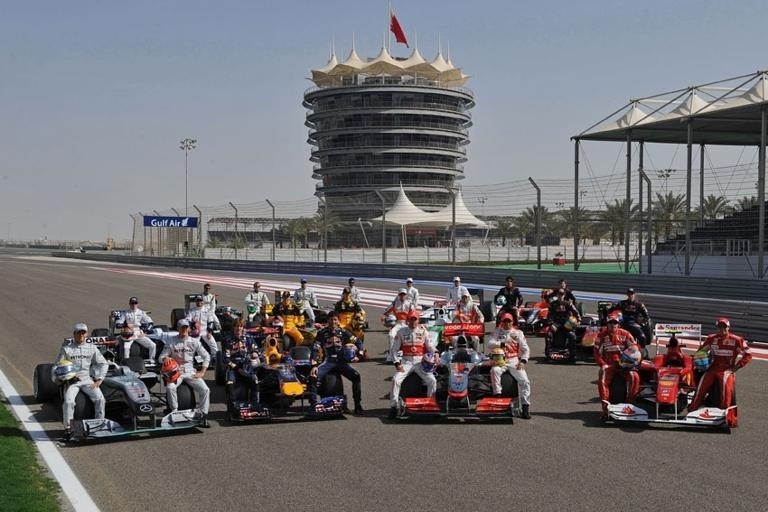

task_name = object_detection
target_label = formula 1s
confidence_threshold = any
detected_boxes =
[33,286,737,443]
[592,311,753,429]
[544,287,654,365]
[386,308,532,425]
[380,275,495,343]
[493,274,583,339]
[33,286,369,443]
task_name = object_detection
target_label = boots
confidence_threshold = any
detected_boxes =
[520,404,530,420]
[353,399,364,416]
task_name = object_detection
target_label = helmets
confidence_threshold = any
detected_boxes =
[383,313,397,327]
[160,358,181,383]
[271,321,283,329]
[496,295,506,305]
[50,359,77,384]
[243,354,258,374]
[342,343,359,363]
[294,299,303,310]
[691,349,711,372]
[618,345,643,369]
[120,332,133,342]
[489,347,507,367]
[419,352,440,376]
[189,328,199,337]
[247,304,256,313]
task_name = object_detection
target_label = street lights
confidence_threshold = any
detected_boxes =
[477,195,488,237]
[577,191,587,237]
[657,168,676,241]
[179,138,197,257]
[555,202,564,238]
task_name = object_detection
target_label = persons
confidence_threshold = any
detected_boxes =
[184,284,220,363]
[116,296,157,360]
[685,316,752,412]
[485,311,531,420]
[157,319,211,427]
[543,277,577,307]
[544,288,582,362]
[50,321,110,435]
[613,286,650,351]
[494,276,523,328]
[243,277,318,344]
[446,277,484,350]
[222,318,260,412]
[383,279,419,361]
[335,278,364,337]
[387,309,440,419]
[593,310,642,423]
[307,311,365,415]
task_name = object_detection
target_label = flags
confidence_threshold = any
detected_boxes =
[389,9,409,49]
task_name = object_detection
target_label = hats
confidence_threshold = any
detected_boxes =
[626,287,635,293]
[129,297,137,304]
[606,312,618,323]
[195,295,202,301]
[715,317,731,328]
[176,319,189,326]
[72,323,87,334]
[301,278,306,284]
[453,277,459,282]
[398,277,413,296]
[406,309,419,321]
[500,313,512,322]
